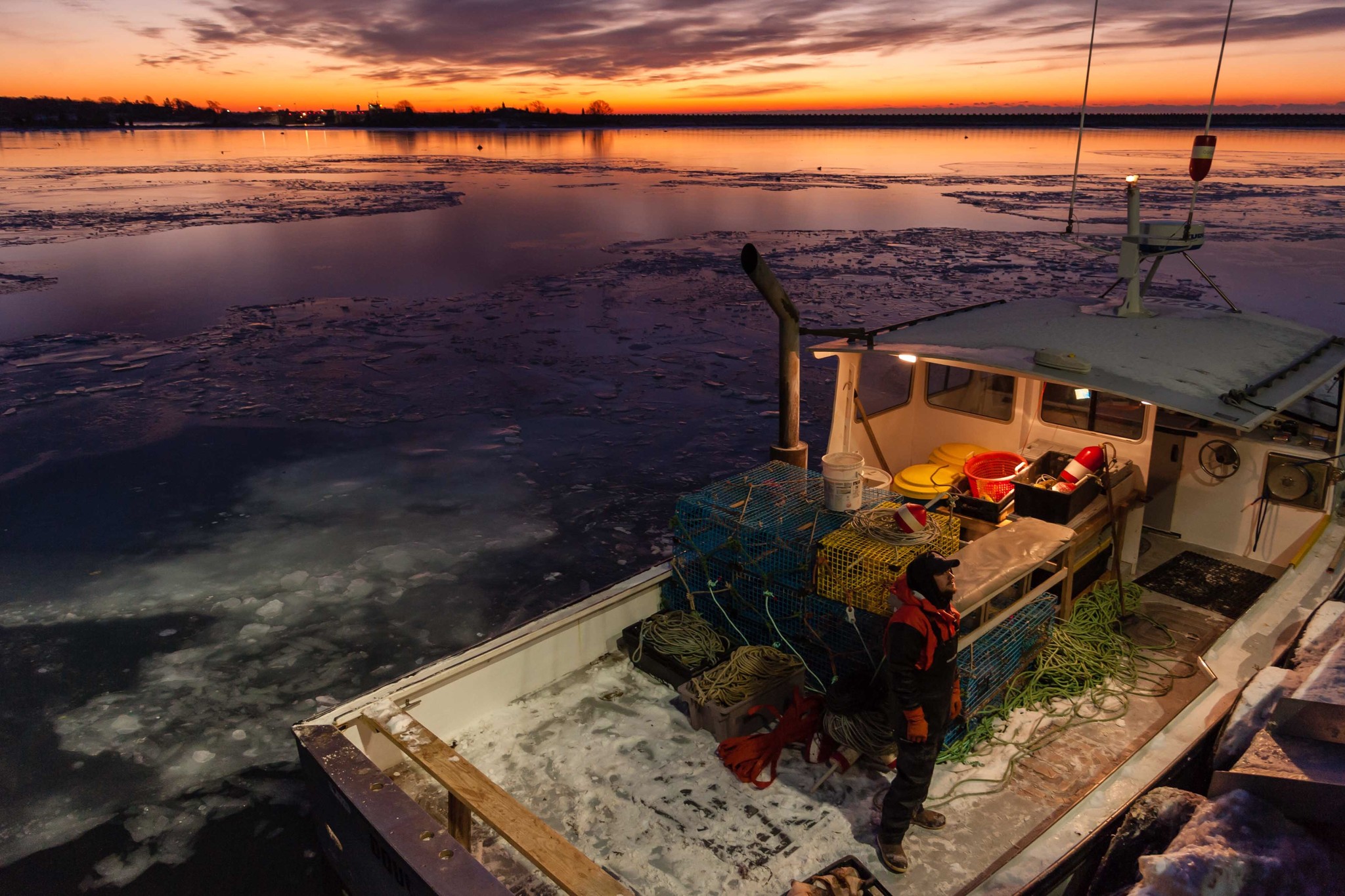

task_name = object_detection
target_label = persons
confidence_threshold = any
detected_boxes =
[876,551,962,872]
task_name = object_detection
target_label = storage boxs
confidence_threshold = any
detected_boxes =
[623,608,738,688]
[677,660,807,741]
[946,438,1133,602]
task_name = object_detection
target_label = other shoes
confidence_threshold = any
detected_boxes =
[909,809,946,830]
[877,834,908,872]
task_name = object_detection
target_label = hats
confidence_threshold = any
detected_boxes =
[931,552,960,575]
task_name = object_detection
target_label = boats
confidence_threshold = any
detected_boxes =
[290,172,1345,896]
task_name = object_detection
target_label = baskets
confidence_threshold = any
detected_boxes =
[963,451,1027,502]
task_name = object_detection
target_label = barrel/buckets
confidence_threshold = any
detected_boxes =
[862,467,892,492]
[821,452,864,512]
